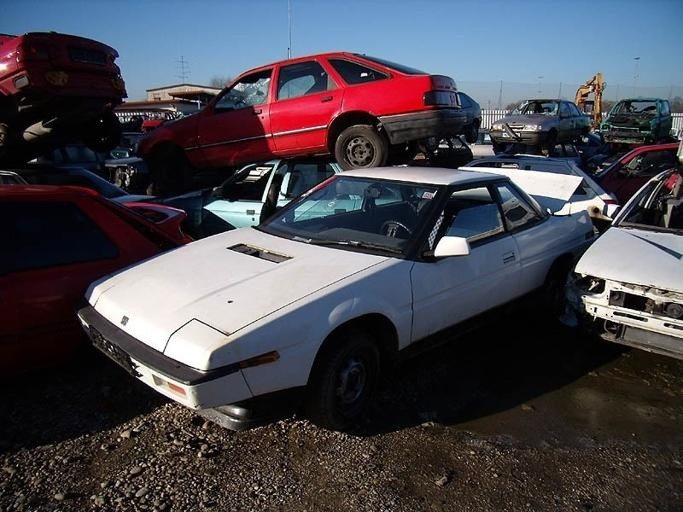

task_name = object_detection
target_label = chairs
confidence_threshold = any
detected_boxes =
[654,177,683,226]
[315,70,342,92]
[622,103,656,112]
[257,170,306,193]
[527,103,557,114]
[366,194,493,237]
